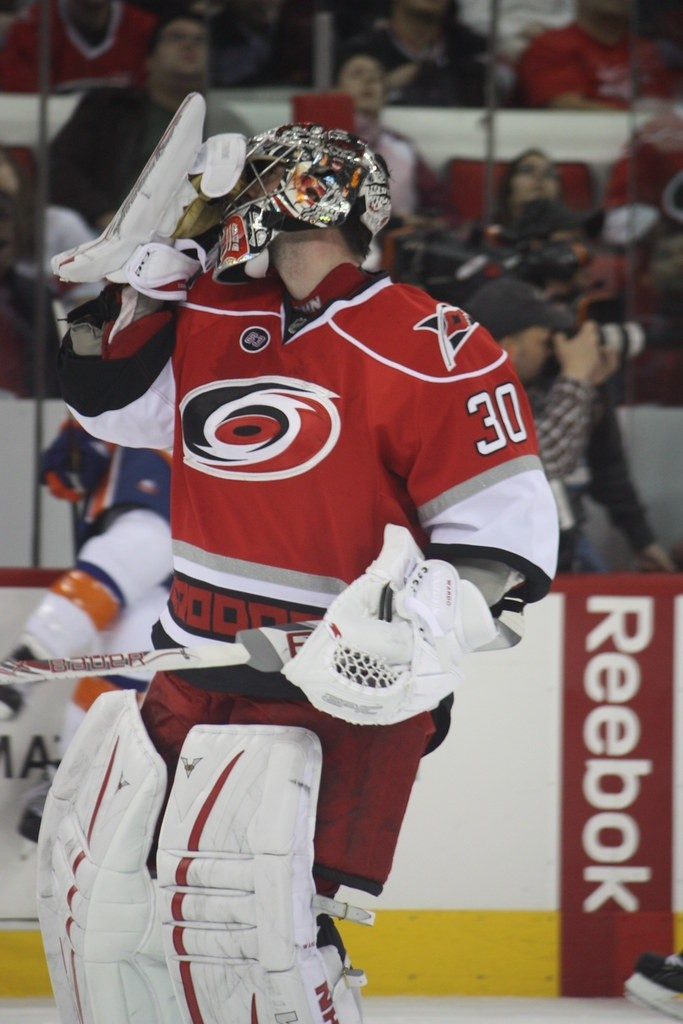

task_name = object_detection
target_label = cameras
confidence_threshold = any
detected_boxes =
[548,312,683,373]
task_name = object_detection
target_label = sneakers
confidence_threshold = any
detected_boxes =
[626,949,682,1021]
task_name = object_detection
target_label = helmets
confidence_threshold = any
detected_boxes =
[213,120,391,285]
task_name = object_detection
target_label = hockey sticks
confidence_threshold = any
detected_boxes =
[1,607,530,689]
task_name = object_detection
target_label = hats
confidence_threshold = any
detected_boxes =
[466,279,576,339]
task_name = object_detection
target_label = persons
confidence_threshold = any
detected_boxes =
[0,0,683,1023]
[37,89,559,1024]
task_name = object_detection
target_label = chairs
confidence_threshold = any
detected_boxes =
[441,156,595,224]
